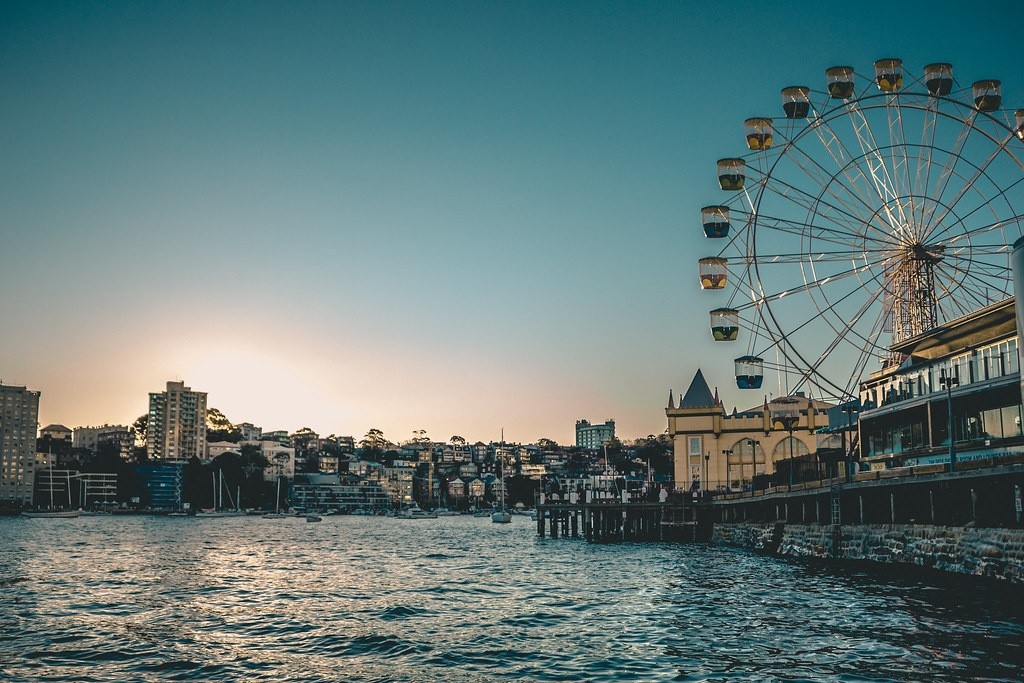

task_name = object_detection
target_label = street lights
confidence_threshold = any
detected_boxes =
[842,405,857,485]
[745,438,760,477]
[939,377,960,472]
[720,449,734,490]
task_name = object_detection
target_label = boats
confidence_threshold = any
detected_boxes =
[349,506,485,522]
[518,506,550,518]
[306,516,322,523]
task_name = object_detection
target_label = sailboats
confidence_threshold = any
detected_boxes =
[490,425,513,523]
[21,442,79,518]
[193,467,247,517]
[261,477,286,519]
